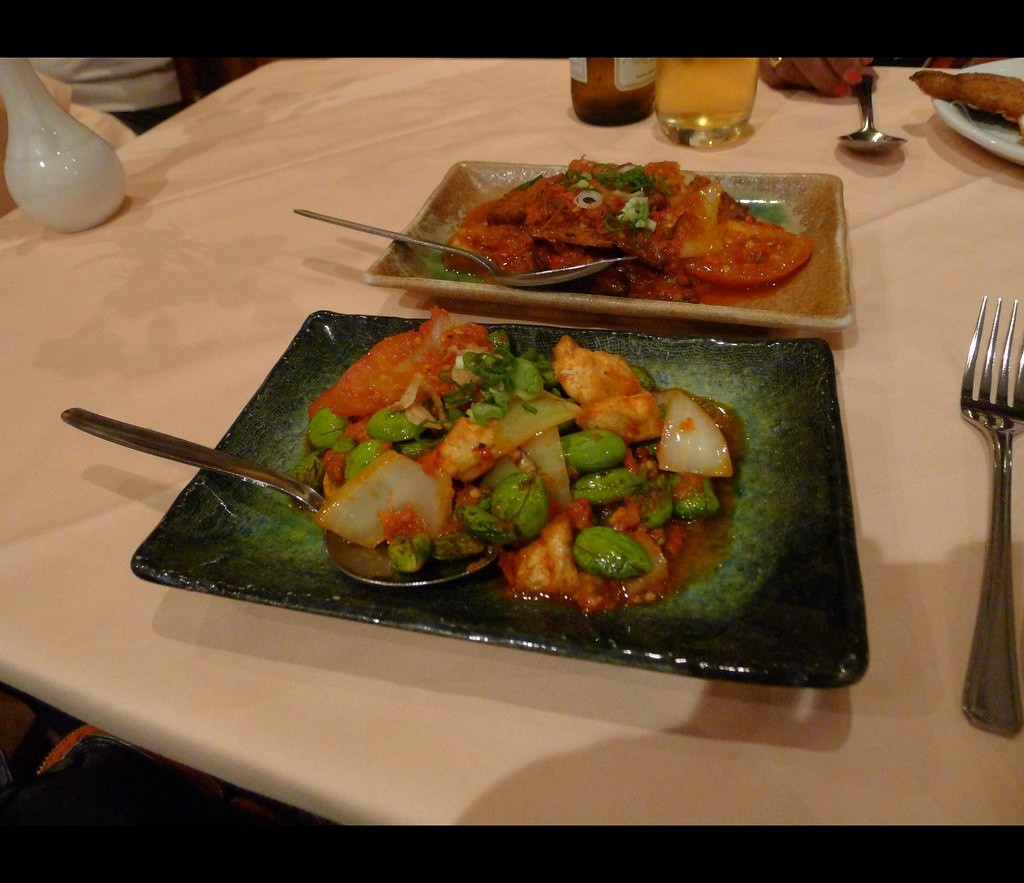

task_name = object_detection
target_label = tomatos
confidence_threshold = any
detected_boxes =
[676,210,814,286]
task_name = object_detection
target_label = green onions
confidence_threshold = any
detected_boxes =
[517,161,673,249]
[410,347,560,447]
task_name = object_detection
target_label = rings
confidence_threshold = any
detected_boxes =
[763,57,782,68]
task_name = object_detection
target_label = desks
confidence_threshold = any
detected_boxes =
[0,59,1024,826]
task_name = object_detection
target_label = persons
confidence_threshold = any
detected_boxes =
[758,57,873,98]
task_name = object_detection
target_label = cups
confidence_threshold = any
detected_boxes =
[652,58,760,150]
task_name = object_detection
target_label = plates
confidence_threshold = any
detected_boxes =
[130,308,869,690]
[930,57,1024,168]
[362,160,853,331]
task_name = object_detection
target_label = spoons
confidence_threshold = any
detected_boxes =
[837,73,908,154]
[293,207,640,288]
[60,406,500,588]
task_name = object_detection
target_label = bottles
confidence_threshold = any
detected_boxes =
[568,58,657,127]
[0,57,132,235]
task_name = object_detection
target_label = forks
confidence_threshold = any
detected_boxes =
[959,296,1024,740]
